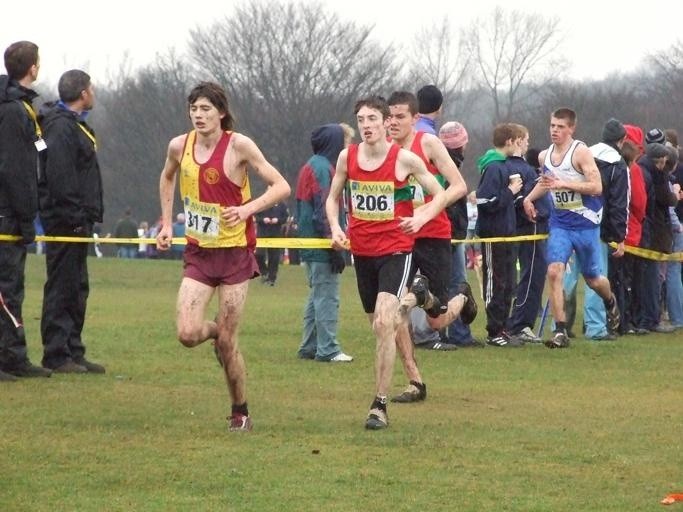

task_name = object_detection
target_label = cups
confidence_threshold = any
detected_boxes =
[509,173,520,184]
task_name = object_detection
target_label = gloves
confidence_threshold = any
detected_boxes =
[327,249,346,275]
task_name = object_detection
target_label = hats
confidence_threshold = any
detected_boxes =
[644,143,672,158]
[601,117,626,141]
[645,127,666,145]
[415,84,443,115]
[437,120,469,151]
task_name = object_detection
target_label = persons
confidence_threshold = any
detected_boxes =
[326,96,451,430]
[156,82,291,435]
[295,123,356,362]
[0,40,53,382]
[388,85,683,351]
[38,69,105,375]
[89,184,353,285]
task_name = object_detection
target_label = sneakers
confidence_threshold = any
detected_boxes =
[211,314,225,369]
[330,353,354,364]
[49,361,88,375]
[0,355,51,382]
[547,292,682,350]
[74,359,106,375]
[226,410,254,435]
[389,379,429,403]
[259,274,276,287]
[363,394,391,430]
[485,330,526,349]
[449,338,485,353]
[409,273,442,318]
[412,335,457,353]
[455,280,478,326]
[507,326,546,348]
